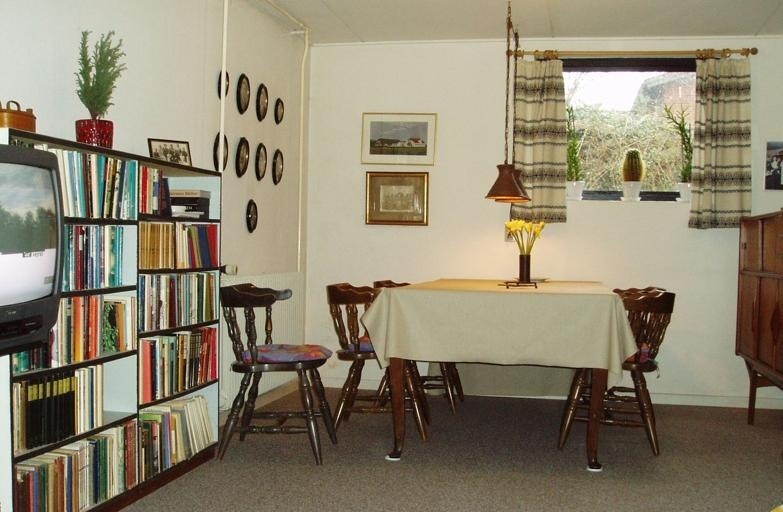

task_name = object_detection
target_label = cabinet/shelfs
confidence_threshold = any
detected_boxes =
[1,125,221,512]
[736,210,783,425]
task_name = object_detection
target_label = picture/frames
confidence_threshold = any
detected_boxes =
[362,113,436,165]
[148,138,192,167]
[367,172,429,225]
[213,70,284,233]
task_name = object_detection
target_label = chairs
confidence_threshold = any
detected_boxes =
[557,287,675,456]
[217,284,337,465]
[326,282,430,440]
[372,280,463,416]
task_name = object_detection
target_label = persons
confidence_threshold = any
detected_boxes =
[766,150,783,186]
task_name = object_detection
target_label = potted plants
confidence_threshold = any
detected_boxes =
[620,150,644,203]
[75,29,127,149]
[566,105,586,200]
[663,102,693,202]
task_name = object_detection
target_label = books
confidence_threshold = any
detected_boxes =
[10,143,218,511]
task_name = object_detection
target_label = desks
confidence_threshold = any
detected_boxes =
[361,278,640,473]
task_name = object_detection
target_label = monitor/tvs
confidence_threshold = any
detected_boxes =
[0,145,65,355]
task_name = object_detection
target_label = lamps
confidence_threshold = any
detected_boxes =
[221,264,237,275]
[495,32,531,204]
[485,0,523,200]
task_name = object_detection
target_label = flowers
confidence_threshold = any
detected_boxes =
[505,211,545,255]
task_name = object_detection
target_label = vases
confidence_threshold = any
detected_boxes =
[520,255,530,282]
